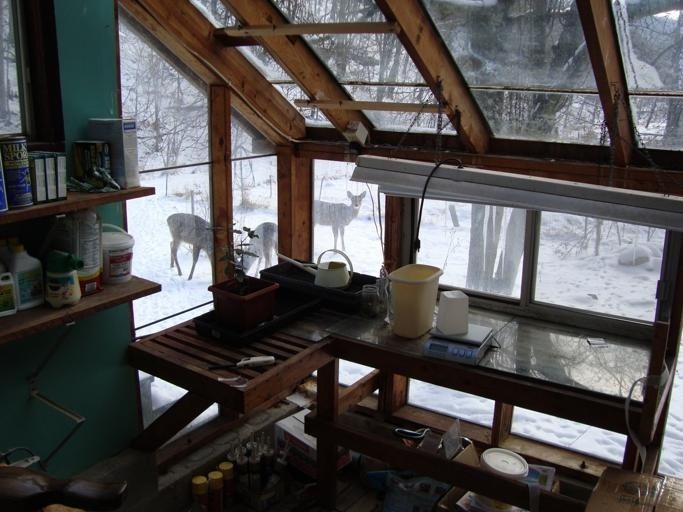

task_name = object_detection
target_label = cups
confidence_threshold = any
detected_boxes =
[361,278,386,318]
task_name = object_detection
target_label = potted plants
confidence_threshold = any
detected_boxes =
[207,220,280,332]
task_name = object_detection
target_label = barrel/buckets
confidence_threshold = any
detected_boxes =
[0,272,17,318]
[96,223,134,285]
[9,242,46,310]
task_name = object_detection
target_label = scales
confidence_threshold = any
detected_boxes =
[423,324,494,366]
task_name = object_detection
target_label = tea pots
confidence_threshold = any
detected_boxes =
[276,249,353,288]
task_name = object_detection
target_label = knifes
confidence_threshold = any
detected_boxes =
[207,355,275,372]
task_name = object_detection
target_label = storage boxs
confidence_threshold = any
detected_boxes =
[584,467,680,512]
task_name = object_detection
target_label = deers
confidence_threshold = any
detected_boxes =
[166,213,213,282]
[239,220,278,276]
[313,190,368,254]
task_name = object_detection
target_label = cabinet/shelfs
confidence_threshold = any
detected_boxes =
[126,301,676,512]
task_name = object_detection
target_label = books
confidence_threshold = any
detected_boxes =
[28,151,67,205]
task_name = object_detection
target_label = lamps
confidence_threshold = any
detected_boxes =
[349,77,682,231]
